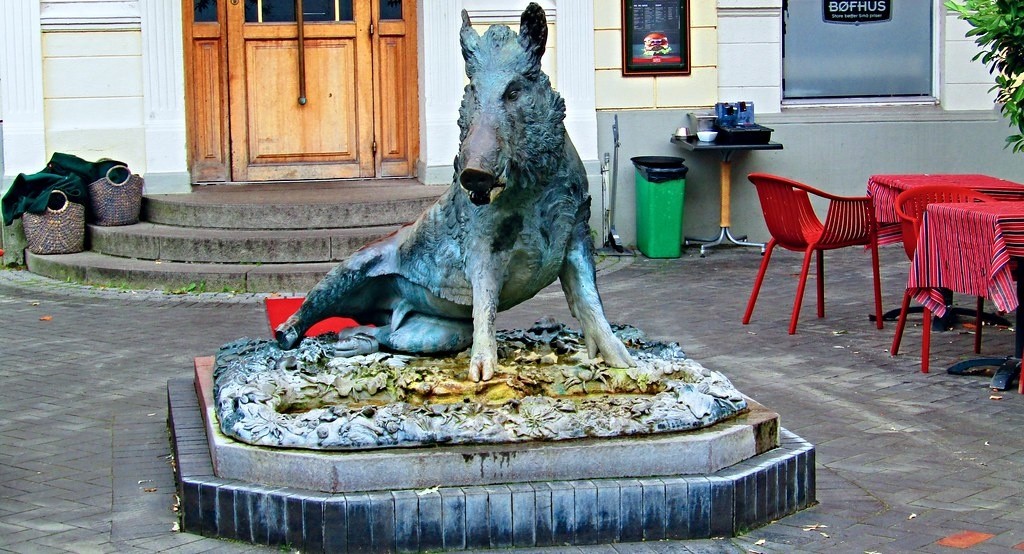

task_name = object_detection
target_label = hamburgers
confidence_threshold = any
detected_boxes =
[644,33,672,56]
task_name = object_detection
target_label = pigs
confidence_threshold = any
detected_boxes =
[273,3,637,382]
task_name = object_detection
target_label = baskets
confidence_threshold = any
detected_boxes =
[88,164,144,226]
[22,189,86,255]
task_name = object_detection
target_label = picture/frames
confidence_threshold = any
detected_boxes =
[620,0,691,77]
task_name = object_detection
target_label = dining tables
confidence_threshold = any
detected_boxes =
[870,175,1024,326]
[926,202,1024,360]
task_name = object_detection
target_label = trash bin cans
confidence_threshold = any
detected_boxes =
[634,162,689,259]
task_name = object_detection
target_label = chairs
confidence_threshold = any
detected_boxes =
[742,172,884,336]
[891,185,998,373]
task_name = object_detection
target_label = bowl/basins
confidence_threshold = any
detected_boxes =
[696,131,718,142]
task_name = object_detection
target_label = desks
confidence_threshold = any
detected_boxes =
[670,136,783,257]
